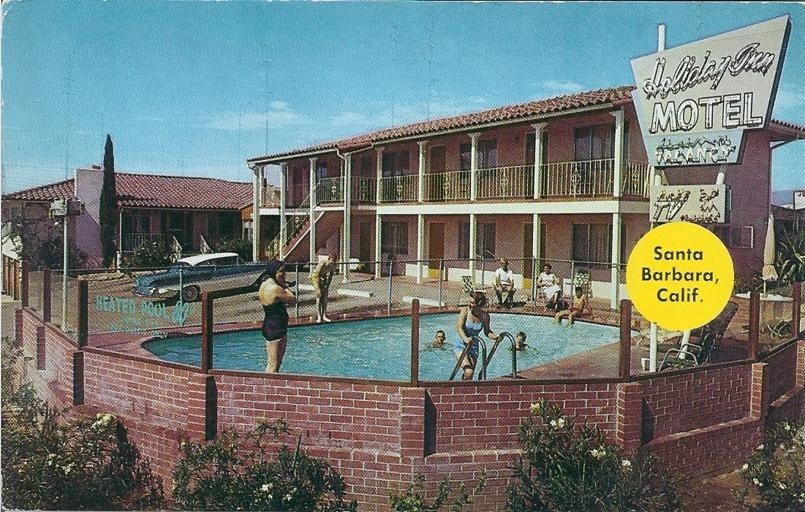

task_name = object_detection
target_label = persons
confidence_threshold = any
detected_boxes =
[536,263,562,304]
[431,330,447,348]
[507,332,530,355]
[310,251,338,324]
[491,257,516,308]
[453,290,504,381]
[257,259,296,373]
[554,286,593,328]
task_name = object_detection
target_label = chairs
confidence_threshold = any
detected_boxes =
[535,274,564,311]
[459,276,488,307]
[656,333,717,371]
[491,270,515,307]
[637,302,739,352]
[566,272,591,302]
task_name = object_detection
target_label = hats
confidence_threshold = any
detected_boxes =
[268,260,283,278]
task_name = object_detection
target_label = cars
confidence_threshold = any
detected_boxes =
[131,250,282,302]
